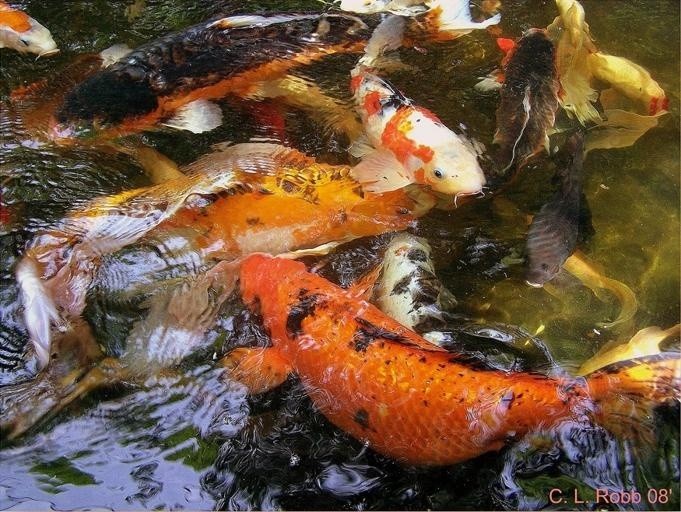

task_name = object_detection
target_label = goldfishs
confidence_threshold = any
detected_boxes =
[0,0,681,512]
